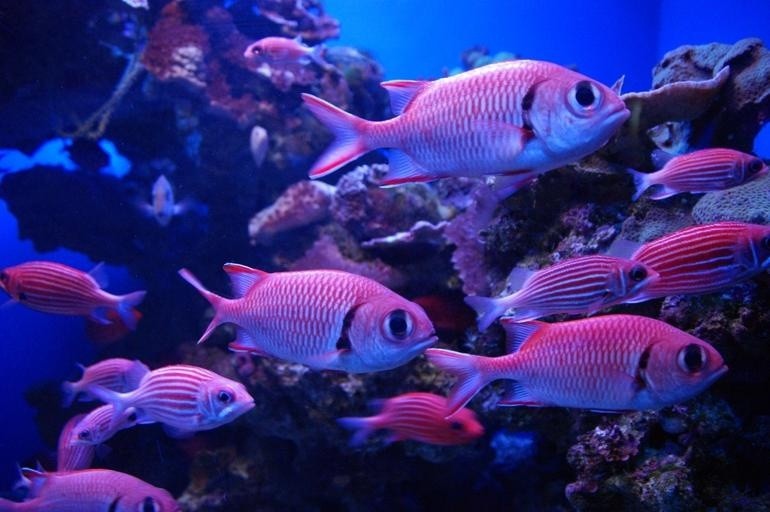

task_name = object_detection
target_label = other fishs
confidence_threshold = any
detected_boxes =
[3,224,765,468]
